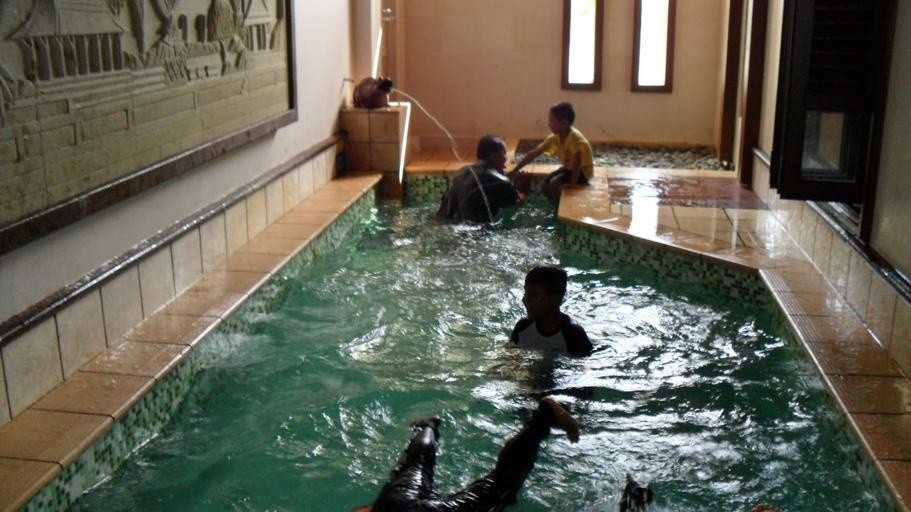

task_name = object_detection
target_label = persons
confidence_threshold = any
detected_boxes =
[509,265,593,358]
[508,102,595,217]
[437,133,526,225]
[750,502,778,512]
[356,397,581,511]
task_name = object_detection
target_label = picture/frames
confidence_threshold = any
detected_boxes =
[0,2,300,259]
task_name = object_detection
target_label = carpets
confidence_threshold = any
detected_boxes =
[511,136,723,169]
